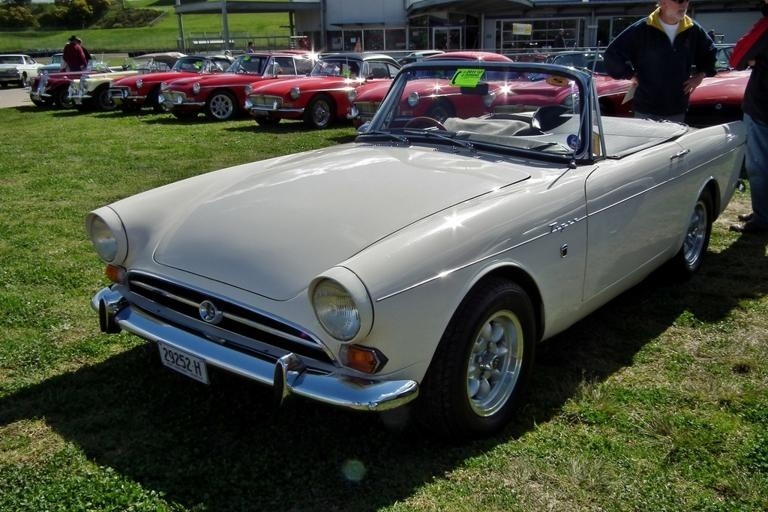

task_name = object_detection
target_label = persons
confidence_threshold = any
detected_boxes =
[602,1,719,124]
[244,40,255,54]
[552,28,567,48]
[63,37,87,72]
[68,36,91,64]
[728,0,767,242]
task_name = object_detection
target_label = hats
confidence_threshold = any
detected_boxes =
[68,35,78,41]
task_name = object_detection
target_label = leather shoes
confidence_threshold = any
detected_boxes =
[730,210,768,234]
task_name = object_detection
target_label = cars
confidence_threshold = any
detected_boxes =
[0,54,46,90]
[685,69,755,123]
[29,50,236,112]
[348,50,531,133]
[158,47,343,122]
[487,52,636,122]
[78,56,754,425]
[35,52,101,76]
[243,52,405,131]
